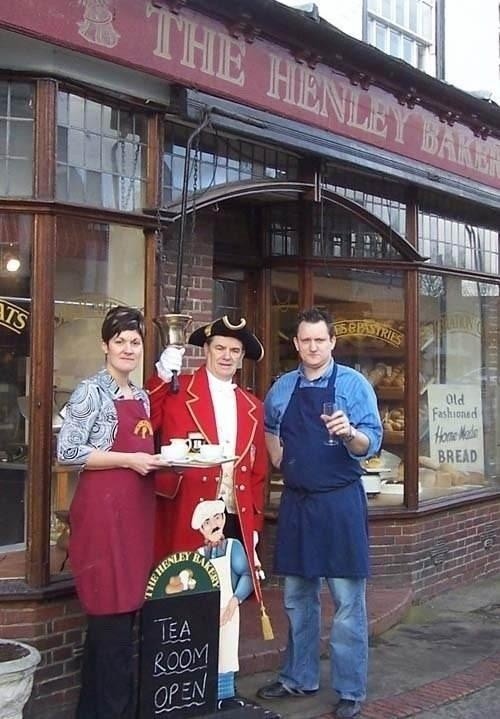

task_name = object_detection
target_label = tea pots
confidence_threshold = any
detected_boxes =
[169,439,193,456]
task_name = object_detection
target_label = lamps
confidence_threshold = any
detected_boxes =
[1,242,21,272]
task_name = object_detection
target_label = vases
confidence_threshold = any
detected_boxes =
[1,638,41,719]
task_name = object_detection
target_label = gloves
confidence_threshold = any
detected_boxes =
[154,347,185,383]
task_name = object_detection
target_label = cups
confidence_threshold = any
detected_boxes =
[160,446,185,460]
[199,445,224,460]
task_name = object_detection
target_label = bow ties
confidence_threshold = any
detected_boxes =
[204,535,225,550]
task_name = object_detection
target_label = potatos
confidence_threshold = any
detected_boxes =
[382,364,406,387]
[379,407,405,432]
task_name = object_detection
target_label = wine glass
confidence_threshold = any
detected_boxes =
[323,402,338,446]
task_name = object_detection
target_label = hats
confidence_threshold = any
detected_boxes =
[191,499,225,530]
[188,315,265,362]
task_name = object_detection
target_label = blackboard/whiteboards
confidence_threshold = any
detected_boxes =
[136,589,221,718]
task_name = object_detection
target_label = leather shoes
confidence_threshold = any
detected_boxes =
[334,700,361,719]
[258,681,319,699]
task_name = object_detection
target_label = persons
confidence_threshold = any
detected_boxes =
[191,500,254,673]
[144,315,270,603]
[256,309,384,719]
[57,305,172,719]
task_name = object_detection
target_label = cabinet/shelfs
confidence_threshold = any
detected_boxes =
[331,320,440,460]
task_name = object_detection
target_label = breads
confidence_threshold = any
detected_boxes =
[397,456,483,488]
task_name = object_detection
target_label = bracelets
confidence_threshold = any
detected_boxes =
[340,425,356,443]
